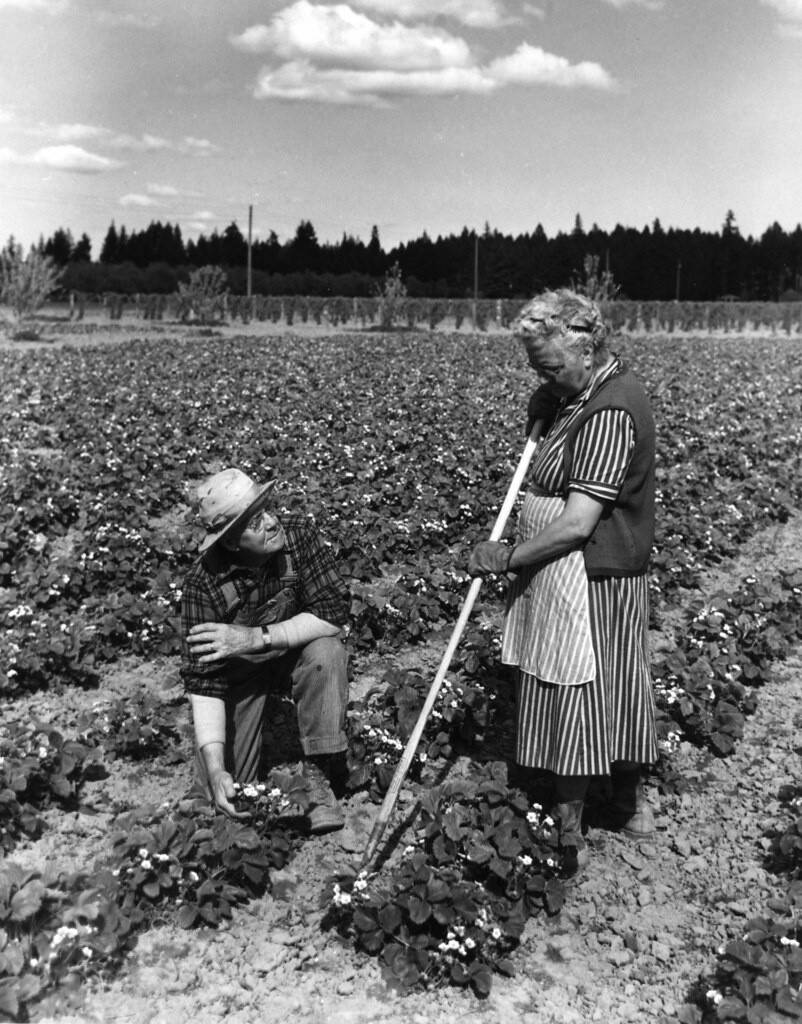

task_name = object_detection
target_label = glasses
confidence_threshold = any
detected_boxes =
[527,348,587,386]
[229,506,276,535]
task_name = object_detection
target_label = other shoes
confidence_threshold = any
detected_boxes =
[525,779,584,880]
[300,757,344,834]
[582,761,655,842]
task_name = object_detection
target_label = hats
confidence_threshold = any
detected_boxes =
[196,468,276,554]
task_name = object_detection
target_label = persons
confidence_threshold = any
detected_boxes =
[180,468,351,832]
[467,288,659,870]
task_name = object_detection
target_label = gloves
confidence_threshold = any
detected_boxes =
[525,381,564,440]
[467,541,517,582]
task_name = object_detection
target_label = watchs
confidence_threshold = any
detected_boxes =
[260,624,271,652]
